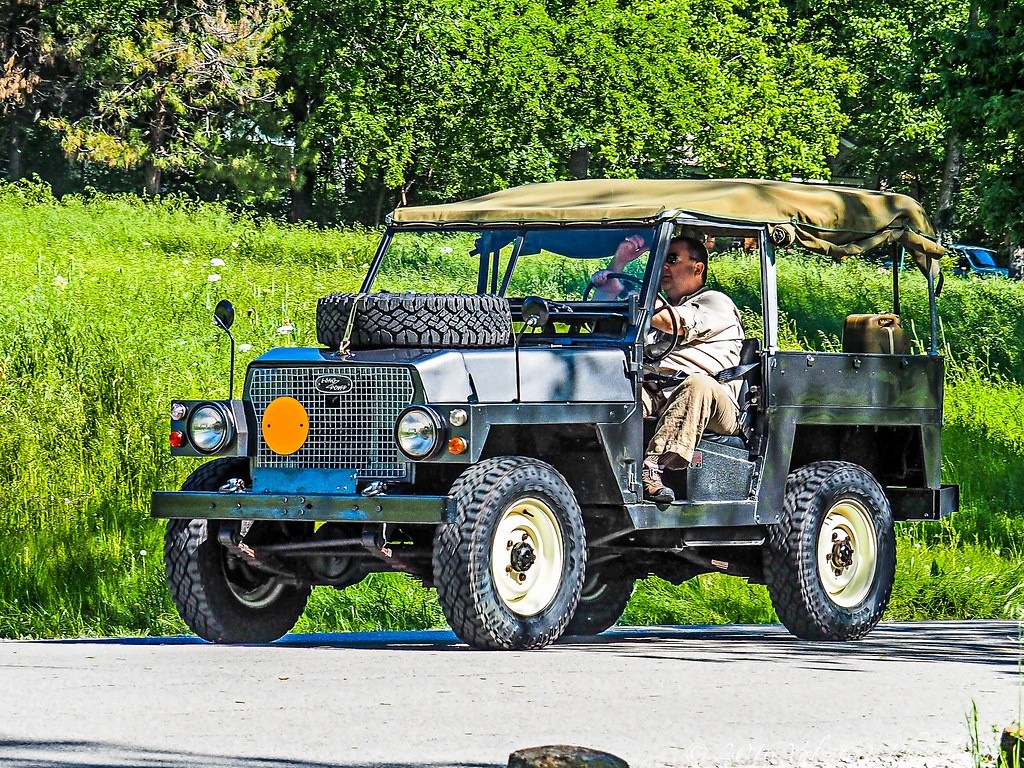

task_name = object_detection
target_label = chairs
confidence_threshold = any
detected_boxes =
[702,338,763,449]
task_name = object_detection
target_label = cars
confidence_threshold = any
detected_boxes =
[882,241,1009,280]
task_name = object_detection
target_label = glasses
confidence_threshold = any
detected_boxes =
[665,252,697,265]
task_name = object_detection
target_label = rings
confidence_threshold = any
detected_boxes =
[625,237,630,242]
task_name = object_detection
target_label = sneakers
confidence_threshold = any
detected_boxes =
[640,468,675,503]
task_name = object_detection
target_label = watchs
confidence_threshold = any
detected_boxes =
[617,280,635,298]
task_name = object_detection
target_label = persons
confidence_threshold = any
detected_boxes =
[589,235,749,504]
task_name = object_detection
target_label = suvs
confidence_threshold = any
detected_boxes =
[145,177,962,650]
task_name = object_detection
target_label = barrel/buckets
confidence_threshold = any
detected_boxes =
[843,313,908,354]
